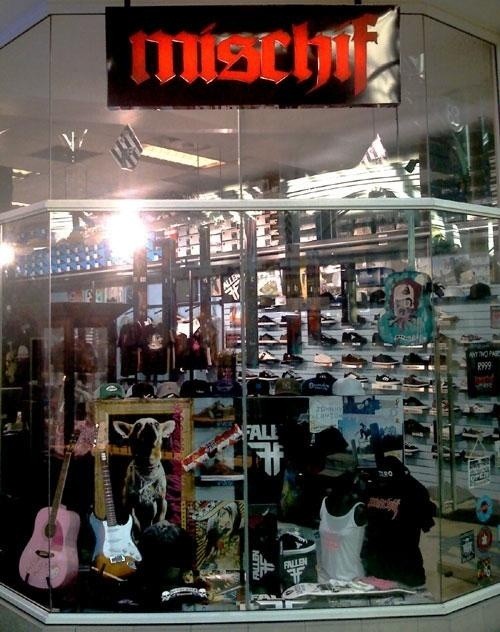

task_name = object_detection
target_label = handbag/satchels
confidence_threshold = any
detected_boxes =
[51,338,98,373]
[54,389,95,456]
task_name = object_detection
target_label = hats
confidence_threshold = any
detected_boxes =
[467,283,491,299]
[318,453,357,478]
[376,456,405,483]
[92,378,366,399]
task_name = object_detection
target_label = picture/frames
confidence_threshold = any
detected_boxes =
[89,395,194,531]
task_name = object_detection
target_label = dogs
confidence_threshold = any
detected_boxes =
[111,416,178,532]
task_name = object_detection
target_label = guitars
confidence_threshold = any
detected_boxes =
[88,421,143,580]
[19,428,82,589]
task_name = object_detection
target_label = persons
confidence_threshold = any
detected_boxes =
[317,453,368,580]
[355,455,435,588]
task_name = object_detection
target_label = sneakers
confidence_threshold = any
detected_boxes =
[319,292,341,307]
[280,532,316,557]
[226,313,400,383]
[403,334,500,457]
[439,311,456,321]
[192,401,235,422]
[199,459,244,481]
